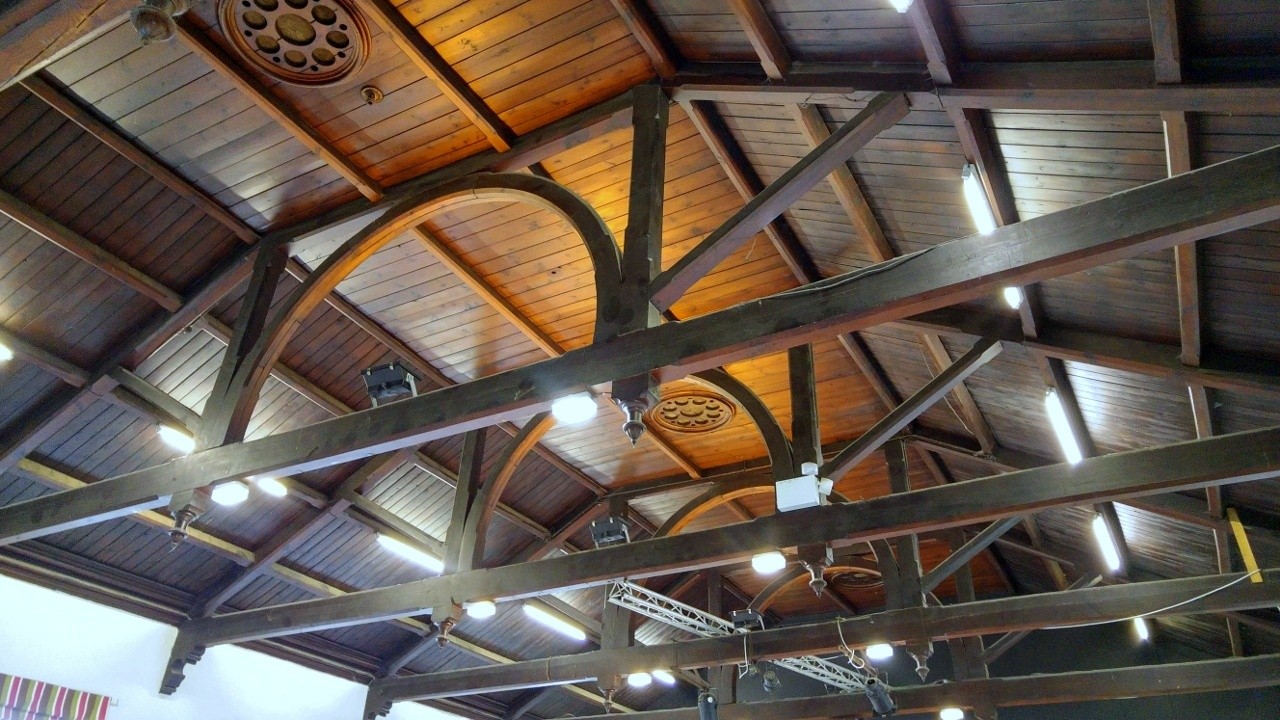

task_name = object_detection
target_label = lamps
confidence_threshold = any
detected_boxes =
[1044,385,1082,467]
[364,365,898,720]
[963,165,1022,312]
[1092,511,1118,573]
